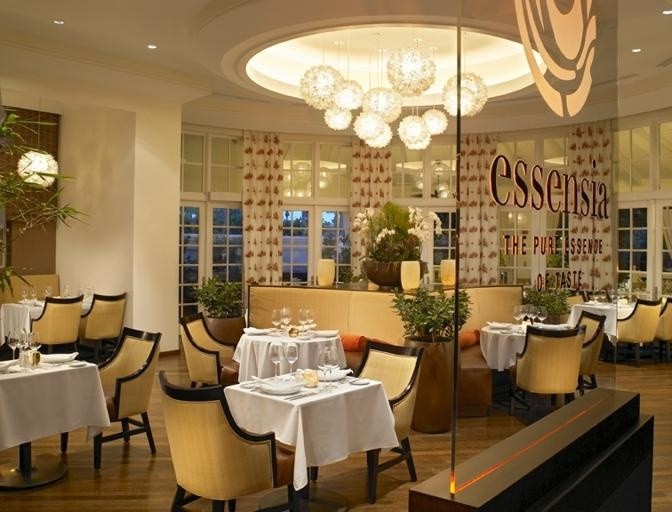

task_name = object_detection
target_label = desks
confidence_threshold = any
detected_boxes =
[222,370,402,512]
[232,329,348,384]
[0,354,97,489]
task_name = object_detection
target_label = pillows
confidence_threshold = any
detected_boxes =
[340,335,366,352]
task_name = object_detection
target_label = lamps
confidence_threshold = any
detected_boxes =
[297,30,492,150]
[18,86,62,187]
[402,261,421,292]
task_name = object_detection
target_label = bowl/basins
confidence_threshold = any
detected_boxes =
[0,361,11,372]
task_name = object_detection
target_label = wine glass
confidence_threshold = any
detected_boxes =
[8,327,42,370]
[316,345,341,394]
[20,283,97,307]
[584,287,651,305]
[266,340,285,376]
[285,342,300,375]
[271,306,317,340]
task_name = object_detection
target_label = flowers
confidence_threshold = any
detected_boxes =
[352,203,444,268]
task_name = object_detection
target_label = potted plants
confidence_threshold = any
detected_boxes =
[392,286,471,433]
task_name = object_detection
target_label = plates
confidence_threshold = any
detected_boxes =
[348,378,371,385]
[308,329,341,337]
[253,372,305,395]
[486,321,571,335]
[243,326,271,336]
[41,351,88,368]
[311,369,354,381]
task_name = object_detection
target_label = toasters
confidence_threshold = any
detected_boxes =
[510,304,549,325]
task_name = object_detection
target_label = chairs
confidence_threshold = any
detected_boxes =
[1,265,127,362]
[507,324,586,424]
[59,326,163,470]
[582,287,612,303]
[617,295,663,368]
[178,311,239,390]
[158,368,296,511]
[570,309,607,396]
[309,340,425,504]
[655,297,672,364]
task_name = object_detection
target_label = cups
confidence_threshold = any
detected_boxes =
[400,260,421,291]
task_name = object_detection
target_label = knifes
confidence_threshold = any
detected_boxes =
[284,392,323,401]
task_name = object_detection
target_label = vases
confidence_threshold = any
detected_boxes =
[362,258,425,291]
